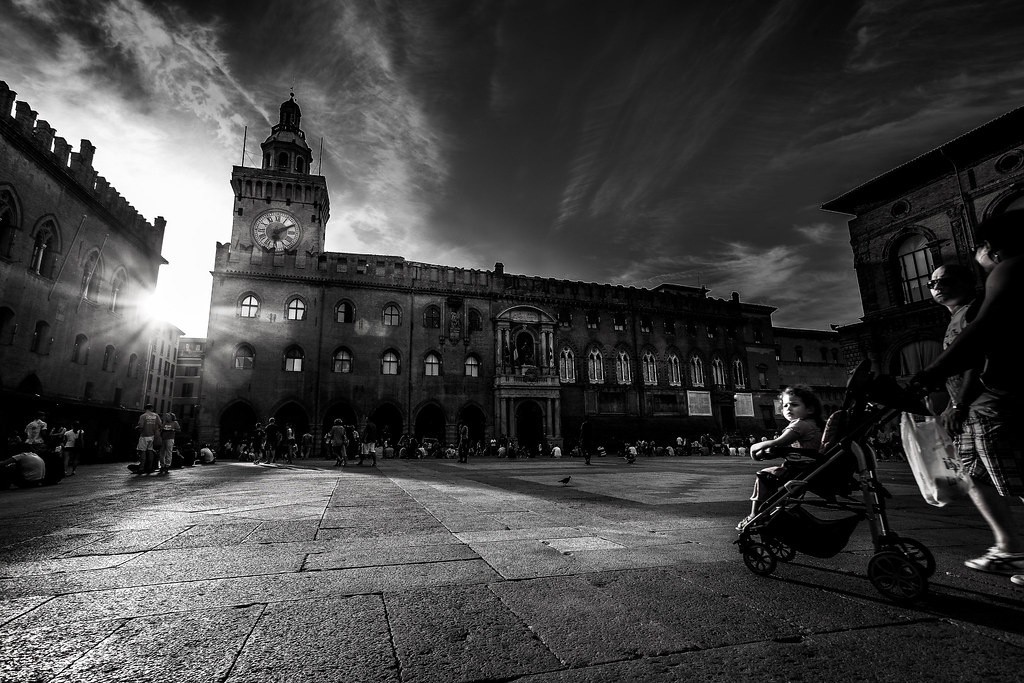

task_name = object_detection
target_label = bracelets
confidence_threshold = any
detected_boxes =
[951,404,971,412]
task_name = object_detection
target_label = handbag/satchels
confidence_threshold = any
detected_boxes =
[899,393,973,508]
[74,430,85,451]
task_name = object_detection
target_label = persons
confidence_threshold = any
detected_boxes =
[51,422,84,476]
[127,445,158,474]
[572,417,786,464]
[915,212,1024,395]
[0,428,46,491]
[87,435,123,461]
[172,417,572,466]
[734,383,828,534]
[158,412,181,473]
[24,410,51,472]
[866,422,909,461]
[136,404,163,474]
[928,263,1023,586]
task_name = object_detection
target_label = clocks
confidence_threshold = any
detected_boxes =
[251,208,304,255]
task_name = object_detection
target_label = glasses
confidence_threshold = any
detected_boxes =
[926,275,961,290]
[969,243,985,255]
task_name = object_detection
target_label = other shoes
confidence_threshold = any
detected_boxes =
[1010,575,1023,586]
[964,546,1024,575]
[735,516,752,531]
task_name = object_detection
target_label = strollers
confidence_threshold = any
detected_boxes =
[733,355,941,605]
